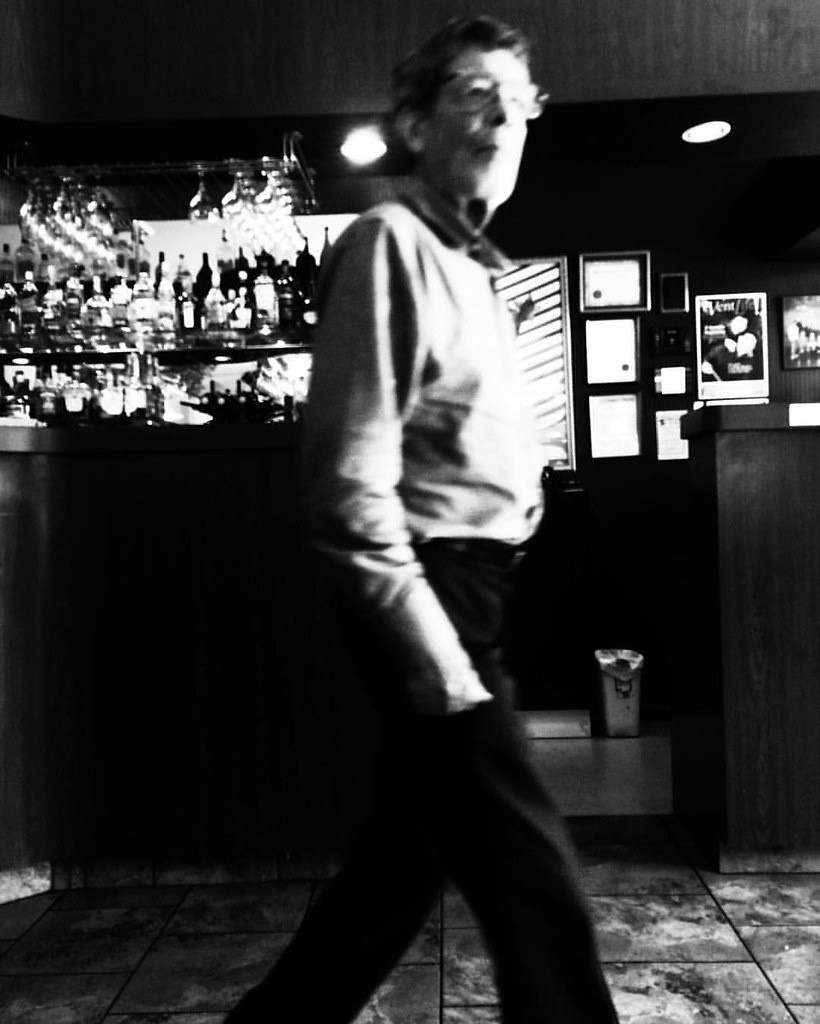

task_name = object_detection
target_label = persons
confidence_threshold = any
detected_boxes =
[225,12,624,1024]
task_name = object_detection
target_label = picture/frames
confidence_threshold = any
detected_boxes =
[579,250,651,312]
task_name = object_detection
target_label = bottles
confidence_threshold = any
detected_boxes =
[10,354,307,421]
[0,238,333,348]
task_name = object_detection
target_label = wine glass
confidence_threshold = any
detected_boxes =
[188,156,304,236]
[19,177,126,255]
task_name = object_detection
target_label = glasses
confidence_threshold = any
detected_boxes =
[443,71,549,120]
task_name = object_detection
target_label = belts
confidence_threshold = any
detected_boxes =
[435,538,526,569]
